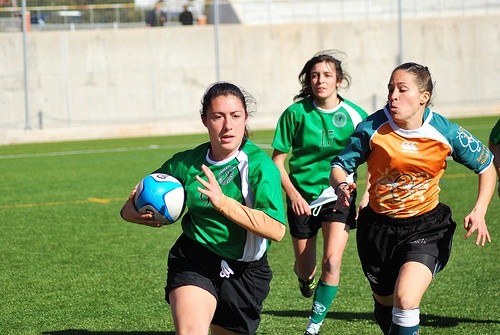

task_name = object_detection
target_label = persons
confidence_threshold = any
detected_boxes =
[270,48,369,335]
[120,80,287,335]
[329,61,498,335]
[178,2,195,26]
[145,0,168,27]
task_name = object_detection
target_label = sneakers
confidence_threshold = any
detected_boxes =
[298,273,317,298]
[303,330,319,335]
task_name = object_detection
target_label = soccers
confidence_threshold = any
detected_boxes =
[135,173,184,225]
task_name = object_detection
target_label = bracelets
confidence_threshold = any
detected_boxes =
[333,181,349,196]
